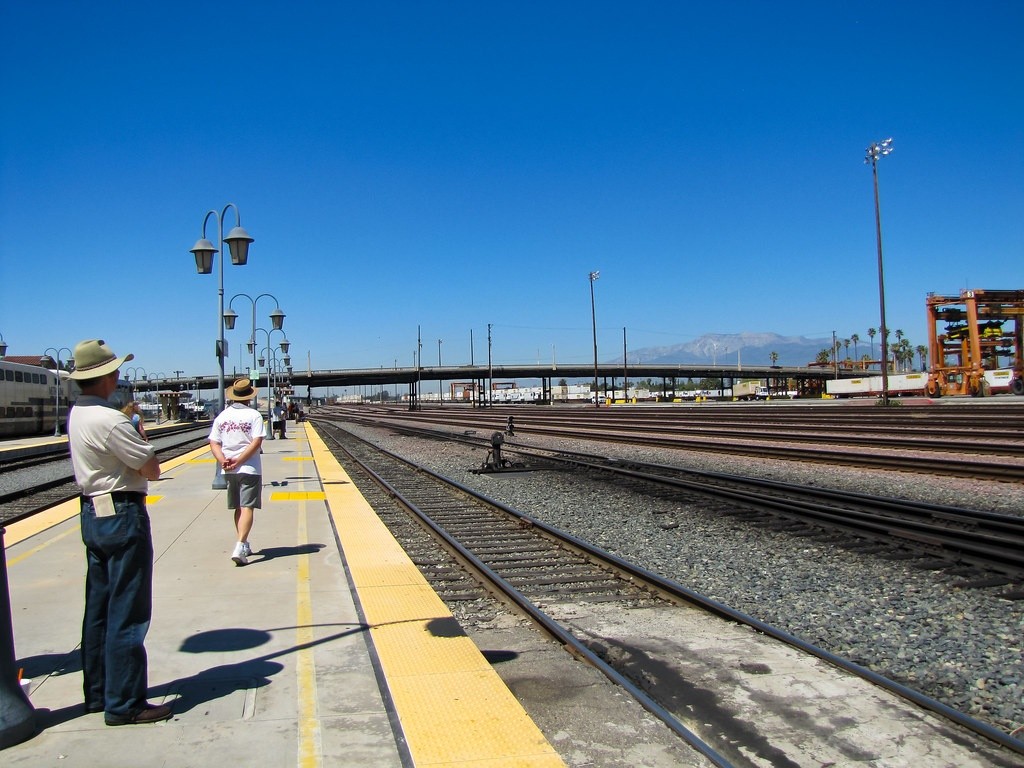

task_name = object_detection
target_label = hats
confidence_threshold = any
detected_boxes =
[227,378,260,400]
[67,339,134,380]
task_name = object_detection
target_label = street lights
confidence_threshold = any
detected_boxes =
[179,380,195,422]
[189,203,256,488]
[123,366,147,402]
[244,328,292,441]
[40,347,77,437]
[586,270,601,405]
[147,372,167,425]
[192,378,201,422]
[438,337,446,407]
[223,293,287,452]
[256,346,294,442]
[864,136,892,407]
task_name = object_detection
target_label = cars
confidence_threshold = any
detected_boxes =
[592,396,606,403]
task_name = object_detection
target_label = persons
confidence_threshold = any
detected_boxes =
[271,402,288,439]
[167,403,172,420]
[180,404,185,409]
[289,403,300,419]
[208,379,267,564]
[122,402,148,443]
[283,402,286,409]
[65,341,172,726]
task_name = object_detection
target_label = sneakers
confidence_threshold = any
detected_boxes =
[105,703,173,724]
[85,704,105,713]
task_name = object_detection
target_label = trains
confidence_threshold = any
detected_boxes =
[139,399,205,413]
[0,358,133,437]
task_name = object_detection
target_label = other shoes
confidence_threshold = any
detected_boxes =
[245,548,252,557]
[231,552,248,567]
[279,436,287,439]
[272,436,276,439]
